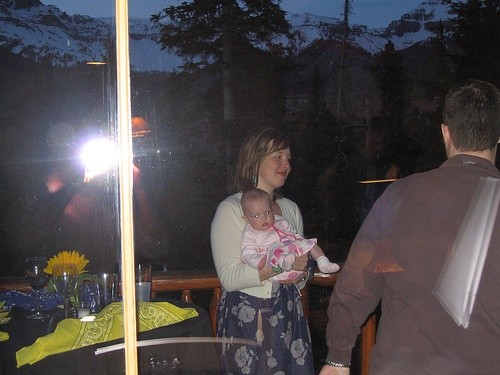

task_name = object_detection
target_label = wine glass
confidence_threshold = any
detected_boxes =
[51,263,78,321]
[24,257,51,320]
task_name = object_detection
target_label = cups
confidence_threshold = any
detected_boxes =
[135,263,152,302]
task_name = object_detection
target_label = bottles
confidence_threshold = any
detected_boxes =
[93,273,119,312]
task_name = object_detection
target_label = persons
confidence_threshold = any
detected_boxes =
[34,116,399,269]
[240,189,339,281]
[318,79,500,375]
[210,126,311,375]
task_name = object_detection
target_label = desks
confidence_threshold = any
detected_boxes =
[0,300,222,375]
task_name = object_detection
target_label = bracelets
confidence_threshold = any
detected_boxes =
[325,359,351,367]
